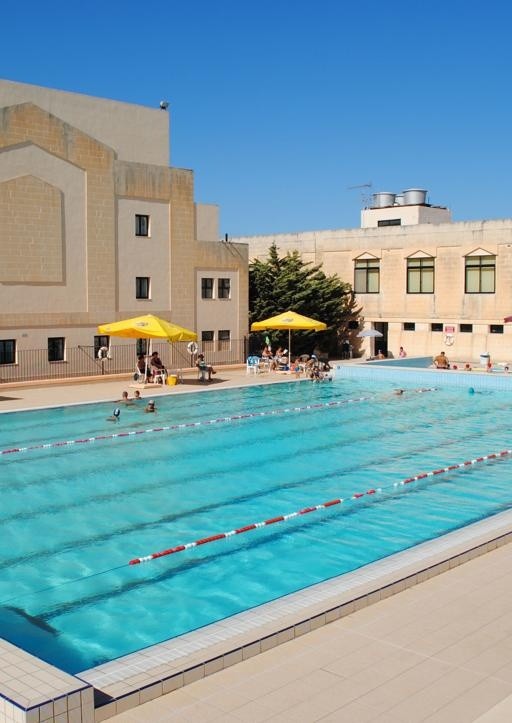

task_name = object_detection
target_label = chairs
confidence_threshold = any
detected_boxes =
[246,356,262,375]
[135,363,179,386]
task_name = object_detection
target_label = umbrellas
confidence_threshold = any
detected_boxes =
[97,312,199,381]
[355,328,383,356]
[250,309,327,369]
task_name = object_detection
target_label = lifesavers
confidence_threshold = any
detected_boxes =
[98,347,111,362]
[187,341,199,355]
[444,333,454,346]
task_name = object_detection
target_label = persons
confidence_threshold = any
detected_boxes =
[452,365,457,369]
[398,346,406,358]
[365,350,384,361]
[195,352,217,382]
[116,391,137,409]
[133,353,154,383]
[504,366,509,373]
[143,399,157,413]
[132,390,142,400]
[433,351,449,368]
[151,351,169,383]
[464,364,471,370]
[487,363,492,372]
[254,331,355,384]
[108,407,120,422]
[447,363,450,368]
